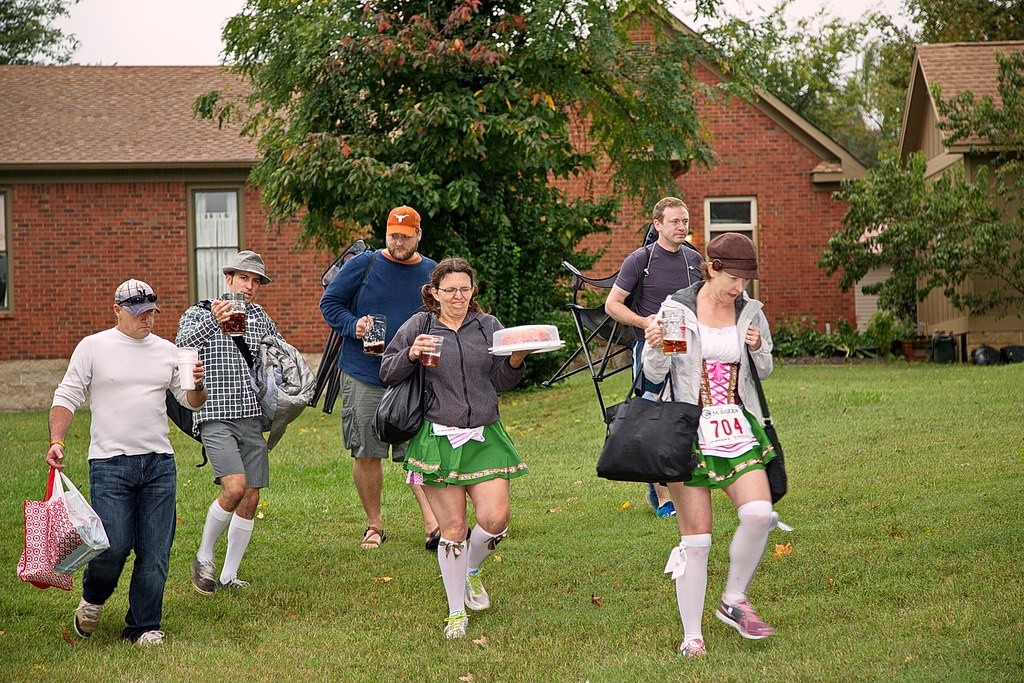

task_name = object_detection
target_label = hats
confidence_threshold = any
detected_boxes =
[387,205,421,237]
[114,278,161,318]
[222,250,273,285]
[706,232,760,280]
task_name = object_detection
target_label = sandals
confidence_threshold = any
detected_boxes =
[360,527,386,549]
[425,527,471,549]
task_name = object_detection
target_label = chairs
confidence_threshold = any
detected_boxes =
[540,221,708,433]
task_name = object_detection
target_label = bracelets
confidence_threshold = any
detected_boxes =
[50,441,67,448]
[193,381,205,391]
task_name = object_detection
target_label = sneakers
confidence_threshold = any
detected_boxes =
[462,567,491,611]
[218,576,250,590]
[717,594,775,641]
[191,553,219,595]
[74,596,103,638]
[444,614,469,640]
[679,640,707,658]
[134,629,165,647]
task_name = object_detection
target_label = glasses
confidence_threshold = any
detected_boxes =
[438,287,473,295]
[118,293,158,306]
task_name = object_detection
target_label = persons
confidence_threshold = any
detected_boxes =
[176,251,287,595]
[320,204,440,551]
[46,277,208,649]
[603,198,710,516]
[379,257,542,640]
[636,230,777,660]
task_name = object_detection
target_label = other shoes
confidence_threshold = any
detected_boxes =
[656,502,677,517]
[647,481,660,511]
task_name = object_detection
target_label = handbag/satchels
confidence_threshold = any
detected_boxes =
[596,363,703,482]
[16,466,111,591]
[761,424,788,506]
[372,313,433,444]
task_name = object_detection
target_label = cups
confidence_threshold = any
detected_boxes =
[421,335,444,368]
[174,346,198,391]
[361,314,387,357]
[221,292,246,337]
[656,309,687,355]
